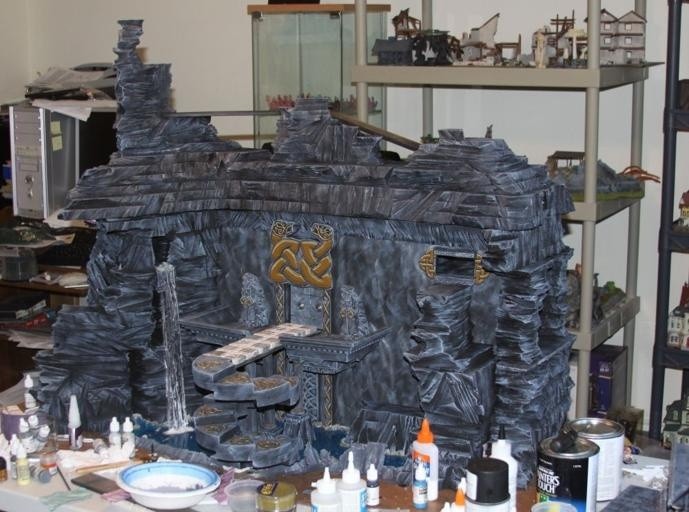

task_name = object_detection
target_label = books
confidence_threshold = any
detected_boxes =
[0,296,58,330]
[52,86,119,112]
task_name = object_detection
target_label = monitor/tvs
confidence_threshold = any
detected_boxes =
[75,108,118,184]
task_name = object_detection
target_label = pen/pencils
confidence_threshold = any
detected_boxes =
[57,467,71,490]
[77,461,130,473]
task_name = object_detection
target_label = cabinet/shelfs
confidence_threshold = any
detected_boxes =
[0,268,87,392]
[645,0,689,440]
[353,1,648,424]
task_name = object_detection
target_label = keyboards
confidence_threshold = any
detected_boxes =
[36,230,96,267]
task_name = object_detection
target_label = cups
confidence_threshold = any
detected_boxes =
[3,249,38,279]
[256,482,299,512]
[223,479,263,511]
[533,500,578,512]
[20,371,41,408]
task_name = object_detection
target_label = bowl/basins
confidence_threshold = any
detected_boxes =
[119,461,222,512]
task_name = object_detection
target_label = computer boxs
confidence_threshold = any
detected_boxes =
[9,101,75,220]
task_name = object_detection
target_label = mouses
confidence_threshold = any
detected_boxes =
[58,272,88,286]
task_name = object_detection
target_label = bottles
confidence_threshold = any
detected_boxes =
[438,475,466,511]
[464,458,511,511]
[0,415,58,486]
[412,418,441,499]
[90,415,135,456]
[336,452,370,511]
[310,467,340,512]
[489,425,519,508]
[366,463,380,507]
[65,395,84,448]
[412,462,429,509]
